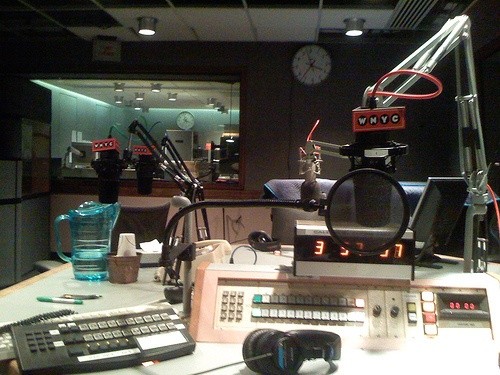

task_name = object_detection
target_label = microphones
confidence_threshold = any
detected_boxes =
[301,143,321,213]
[91,137,124,204]
[133,146,156,194]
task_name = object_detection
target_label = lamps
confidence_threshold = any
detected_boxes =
[168,92,178,102]
[133,104,149,113]
[115,95,124,104]
[138,16,158,36]
[206,98,229,116]
[115,82,127,92]
[343,17,366,37]
[135,91,145,101]
[150,83,161,93]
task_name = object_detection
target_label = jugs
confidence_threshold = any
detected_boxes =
[54,200,121,282]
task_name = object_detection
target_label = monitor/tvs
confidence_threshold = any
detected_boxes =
[407,177,470,248]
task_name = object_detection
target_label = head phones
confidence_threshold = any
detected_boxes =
[243,329,341,375]
[248,229,282,253]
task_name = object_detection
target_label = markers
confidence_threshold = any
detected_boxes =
[65,293,103,299]
[37,297,83,305]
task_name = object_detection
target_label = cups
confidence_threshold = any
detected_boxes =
[104,251,142,283]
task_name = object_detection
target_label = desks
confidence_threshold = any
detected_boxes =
[0,251,500,375]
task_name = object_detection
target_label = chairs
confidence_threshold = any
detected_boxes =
[34,202,171,274]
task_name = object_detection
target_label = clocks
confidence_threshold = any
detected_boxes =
[292,45,333,86]
[175,111,195,130]
